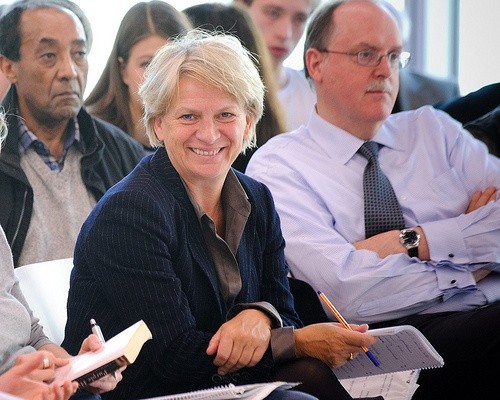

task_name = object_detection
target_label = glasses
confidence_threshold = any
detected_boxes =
[320,48,412,70]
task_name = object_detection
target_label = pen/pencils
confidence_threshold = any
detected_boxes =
[317,290,384,372]
[90,319,105,345]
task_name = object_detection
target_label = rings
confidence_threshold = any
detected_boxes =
[43,358,49,369]
[348,353,353,361]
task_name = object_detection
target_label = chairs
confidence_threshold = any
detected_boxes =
[13,256,74,350]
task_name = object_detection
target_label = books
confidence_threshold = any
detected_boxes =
[49,320,152,389]
[331,325,444,399]
[140,382,301,400]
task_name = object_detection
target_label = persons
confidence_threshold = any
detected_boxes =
[233,0,315,132]
[245,0,500,400]
[60,28,378,400]
[0,0,147,268]
[0,224,127,400]
[83,0,193,147]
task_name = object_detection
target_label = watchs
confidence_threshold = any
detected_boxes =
[399,227,420,259]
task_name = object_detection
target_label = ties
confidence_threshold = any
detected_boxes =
[355,140,405,242]
